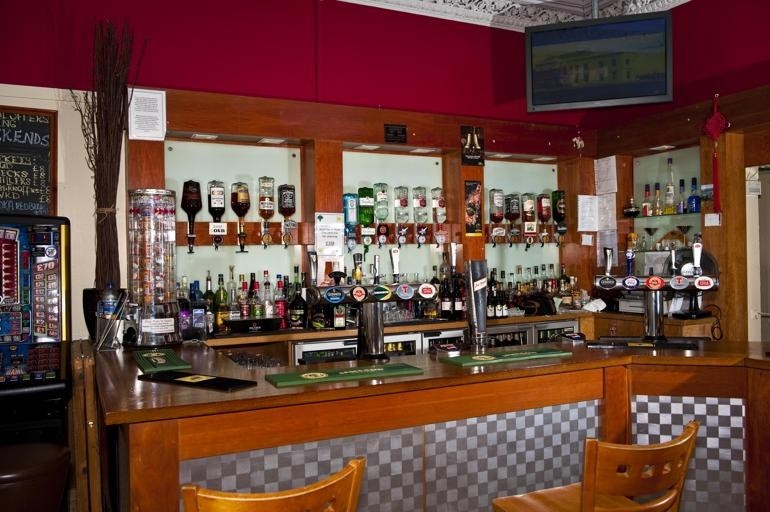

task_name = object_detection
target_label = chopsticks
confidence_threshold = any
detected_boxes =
[97,291,129,350]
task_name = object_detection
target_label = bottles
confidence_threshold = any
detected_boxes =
[623,197,641,218]
[677,179,687,214]
[278,185,296,248]
[303,351,344,361]
[344,183,447,255]
[488,328,571,347]
[96,287,127,351]
[687,177,701,214]
[652,182,664,216]
[693,233,697,243]
[488,187,567,250]
[662,157,677,216]
[218,350,283,368]
[636,236,645,251]
[384,342,413,355]
[177,253,467,340]
[487,262,575,318]
[642,184,653,217]
[259,177,275,248]
[180,180,202,253]
[207,180,226,251]
[231,182,250,253]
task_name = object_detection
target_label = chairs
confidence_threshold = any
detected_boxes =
[493,419,701,512]
[177,453,366,512]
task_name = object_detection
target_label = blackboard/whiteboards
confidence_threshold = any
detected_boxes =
[0,104,58,217]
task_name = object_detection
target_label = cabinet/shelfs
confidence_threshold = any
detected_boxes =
[594,312,716,344]
[628,203,704,258]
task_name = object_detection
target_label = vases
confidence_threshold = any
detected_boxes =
[83,288,127,344]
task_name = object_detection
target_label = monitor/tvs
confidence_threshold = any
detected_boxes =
[525,11,672,113]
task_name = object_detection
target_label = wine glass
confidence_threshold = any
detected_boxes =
[643,227,658,250]
[677,225,692,249]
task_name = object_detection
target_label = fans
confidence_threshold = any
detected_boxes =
[658,246,722,321]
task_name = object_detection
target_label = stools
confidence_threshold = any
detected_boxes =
[1,438,73,511]
